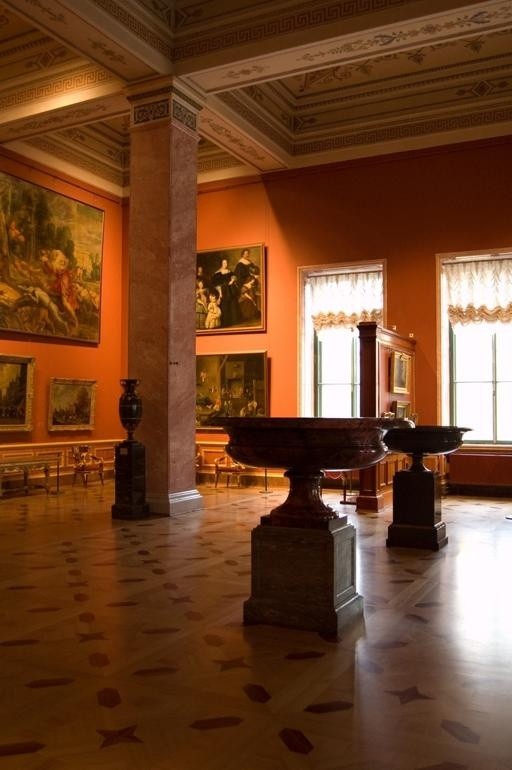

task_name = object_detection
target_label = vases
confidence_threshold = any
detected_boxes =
[117,375,144,445]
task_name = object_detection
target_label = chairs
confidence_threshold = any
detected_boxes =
[71,445,104,485]
[195,443,203,483]
[212,449,244,489]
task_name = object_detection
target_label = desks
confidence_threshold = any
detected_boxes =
[0,456,59,495]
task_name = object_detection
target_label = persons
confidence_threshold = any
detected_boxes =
[193,248,258,329]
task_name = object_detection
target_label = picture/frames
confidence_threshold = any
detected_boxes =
[0,166,106,345]
[411,413,419,425]
[46,376,98,434]
[1,354,40,433]
[382,411,395,418]
[389,348,415,397]
[194,349,273,433]
[391,399,412,422]
[194,243,269,337]
